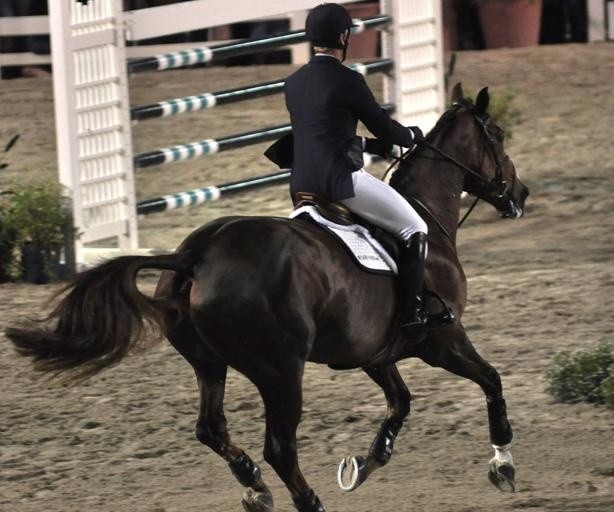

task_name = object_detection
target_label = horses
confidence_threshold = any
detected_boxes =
[0,80,531,512]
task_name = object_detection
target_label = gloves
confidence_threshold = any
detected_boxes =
[363,134,394,160]
[405,125,424,147]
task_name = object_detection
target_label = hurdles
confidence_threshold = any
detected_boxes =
[13,0,444,278]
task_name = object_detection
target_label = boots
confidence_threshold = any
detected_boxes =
[399,230,456,340]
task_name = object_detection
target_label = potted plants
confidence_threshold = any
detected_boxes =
[1,185,76,283]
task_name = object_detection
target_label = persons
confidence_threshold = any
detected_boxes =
[261,3,453,328]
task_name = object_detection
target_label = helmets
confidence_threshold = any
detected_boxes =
[305,3,360,47]
